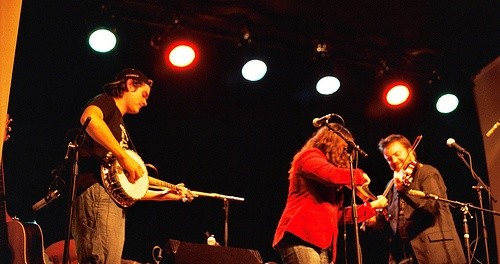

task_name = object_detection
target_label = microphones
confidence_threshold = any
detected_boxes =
[32,188,59,210]
[65,142,72,158]
[312,113,334,127]
[447,138,469,155]
[408,189,438,199]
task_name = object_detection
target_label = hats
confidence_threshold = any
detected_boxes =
[104,69,151,93]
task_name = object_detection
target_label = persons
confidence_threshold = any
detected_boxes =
[65,65,197,263]
[357,133,471,264]
[271,124,389,264]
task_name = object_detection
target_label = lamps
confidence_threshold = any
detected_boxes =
[234,25,271,86]
[375,64,414,111]
[82,4,119,58]
[308,52,342,97]
[423,72,462,117]
[159,26,201,73]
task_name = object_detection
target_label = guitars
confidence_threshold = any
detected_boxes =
[101,149,245,208]
[0,113,46,264]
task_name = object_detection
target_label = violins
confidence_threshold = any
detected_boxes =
[394,160,417,192]
[344,184,394,221]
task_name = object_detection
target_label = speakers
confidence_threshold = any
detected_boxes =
[157,240,263,264]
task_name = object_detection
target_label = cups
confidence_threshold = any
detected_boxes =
[207,237,216,245]
